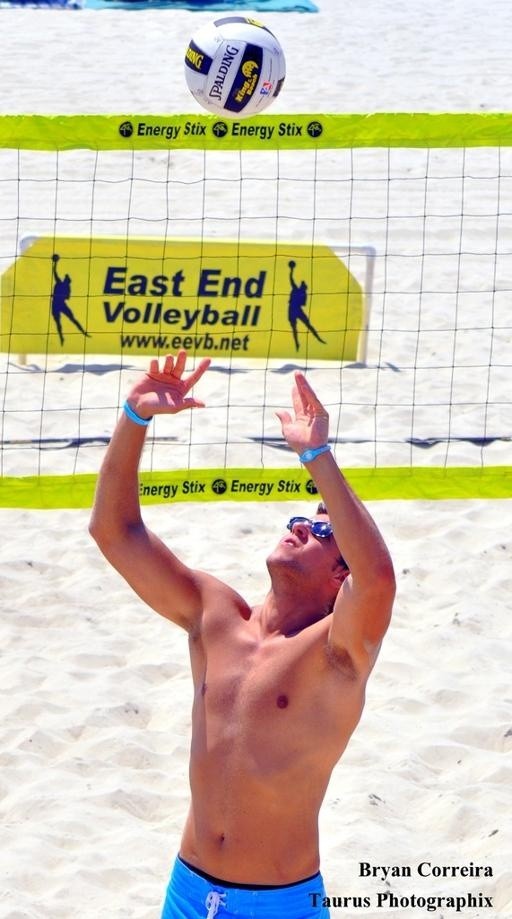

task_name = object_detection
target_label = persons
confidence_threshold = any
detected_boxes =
[51,258,90,347]
[287,258,328,351]
[87,354,396,918]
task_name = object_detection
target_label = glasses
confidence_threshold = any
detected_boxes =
[286,515,335,538]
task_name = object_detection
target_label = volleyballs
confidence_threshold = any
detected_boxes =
[183,18,284,118]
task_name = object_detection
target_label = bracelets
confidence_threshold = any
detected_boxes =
[119,399,151,424]
[297,441,331,462]
